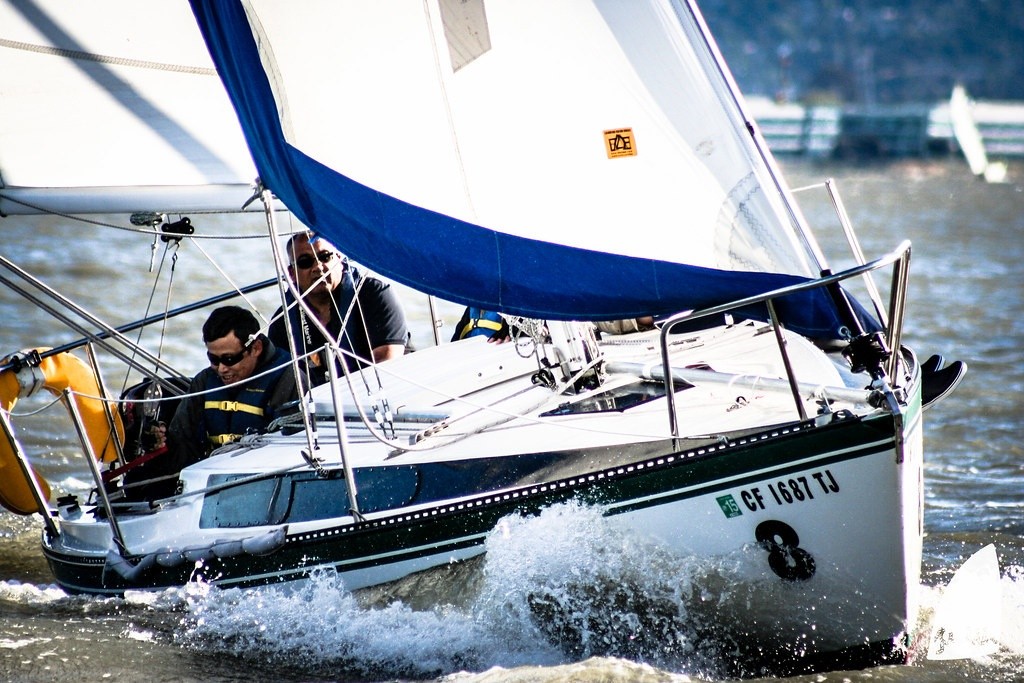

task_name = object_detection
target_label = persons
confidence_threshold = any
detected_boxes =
[266,229,417,387]
[596,244,969,415]
[450,306,532,345]
[141,306,318,467]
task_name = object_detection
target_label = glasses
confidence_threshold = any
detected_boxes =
[207,346,251,367]
[290,250,336,270]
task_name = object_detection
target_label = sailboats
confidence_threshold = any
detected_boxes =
[0,1,971,675]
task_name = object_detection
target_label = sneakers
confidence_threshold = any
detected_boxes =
[922,361,967,411]
[920,355,944,376]
